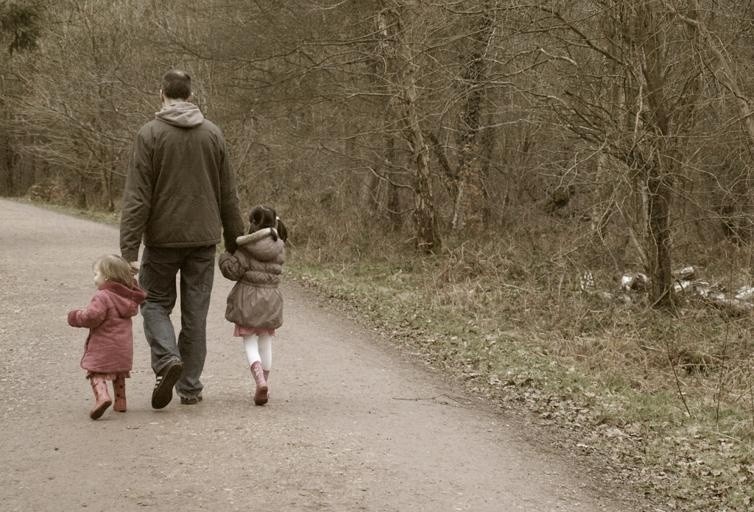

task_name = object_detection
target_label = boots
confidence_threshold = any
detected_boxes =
[113,377,126,413]
[90,374,112,421]
[250,361,269,405]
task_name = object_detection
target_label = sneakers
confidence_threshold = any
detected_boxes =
[181,391,203,405]
[151,359,184,409]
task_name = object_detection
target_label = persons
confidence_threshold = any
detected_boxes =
[68,253,146,421]
[219,202,285,409]
[121,69,245,409]
